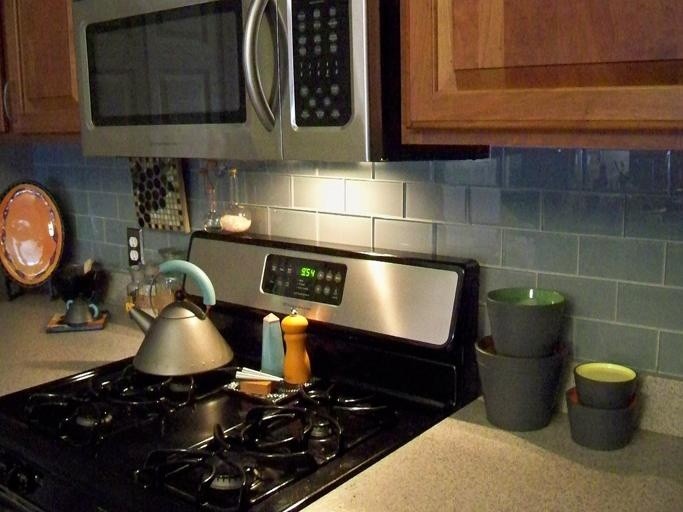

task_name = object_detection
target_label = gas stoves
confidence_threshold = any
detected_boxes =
[0,353,455,512]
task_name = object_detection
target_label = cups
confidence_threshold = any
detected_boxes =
[571,358,642,409]
[473,335,572,433]
[561,385,643,452]
[483,285,566,358]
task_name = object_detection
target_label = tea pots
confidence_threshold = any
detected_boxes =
[123,259,236,378]
[62,296,101,328]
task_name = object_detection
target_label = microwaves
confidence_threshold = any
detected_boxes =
[68,0,495,164]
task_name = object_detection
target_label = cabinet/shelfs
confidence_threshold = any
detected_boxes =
[400,0,683,151]
[0,0,81,135]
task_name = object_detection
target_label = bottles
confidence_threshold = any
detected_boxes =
[279,307,312,386]
[202,167,254,235]
[260,311,284,381]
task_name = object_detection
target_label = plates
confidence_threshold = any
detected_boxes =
[0,179,66,287]
